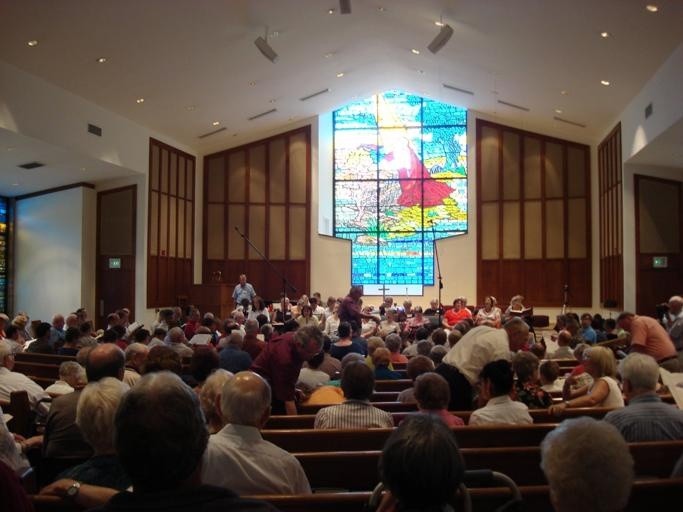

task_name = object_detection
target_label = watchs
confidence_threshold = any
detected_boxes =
[64,478,83,499]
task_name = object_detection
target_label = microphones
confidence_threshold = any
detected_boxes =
[234,225,243,239]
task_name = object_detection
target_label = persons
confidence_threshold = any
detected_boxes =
[0,267,682,506]
